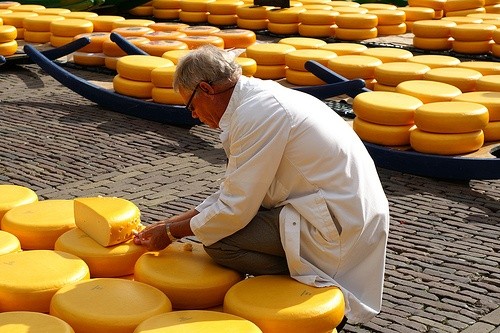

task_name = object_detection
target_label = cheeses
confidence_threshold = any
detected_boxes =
[0,0,500,333]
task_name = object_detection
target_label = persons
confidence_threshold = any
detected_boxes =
[134,44,390,332]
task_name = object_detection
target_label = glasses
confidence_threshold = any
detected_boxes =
[185,84,199,112]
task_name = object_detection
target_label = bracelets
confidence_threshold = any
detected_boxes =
[165,222,177,243]
